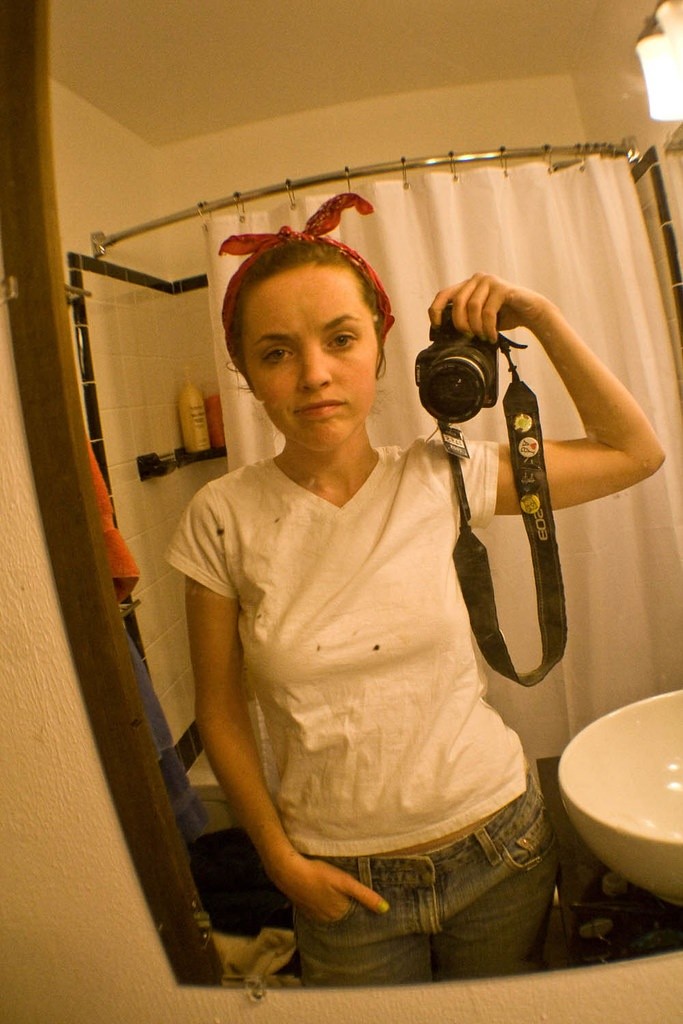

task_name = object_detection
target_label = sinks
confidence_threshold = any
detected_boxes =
[557,695,683,907]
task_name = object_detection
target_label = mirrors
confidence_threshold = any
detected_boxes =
[0,2,683,991]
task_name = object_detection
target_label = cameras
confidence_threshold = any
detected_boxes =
[414,303,499,423]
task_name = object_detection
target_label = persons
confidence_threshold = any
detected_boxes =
[164,192,667,989]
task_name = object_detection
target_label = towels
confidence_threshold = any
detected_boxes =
[83,428,139,607]
[119,620,209,845]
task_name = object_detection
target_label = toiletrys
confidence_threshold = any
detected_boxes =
[175,363,224,453]
